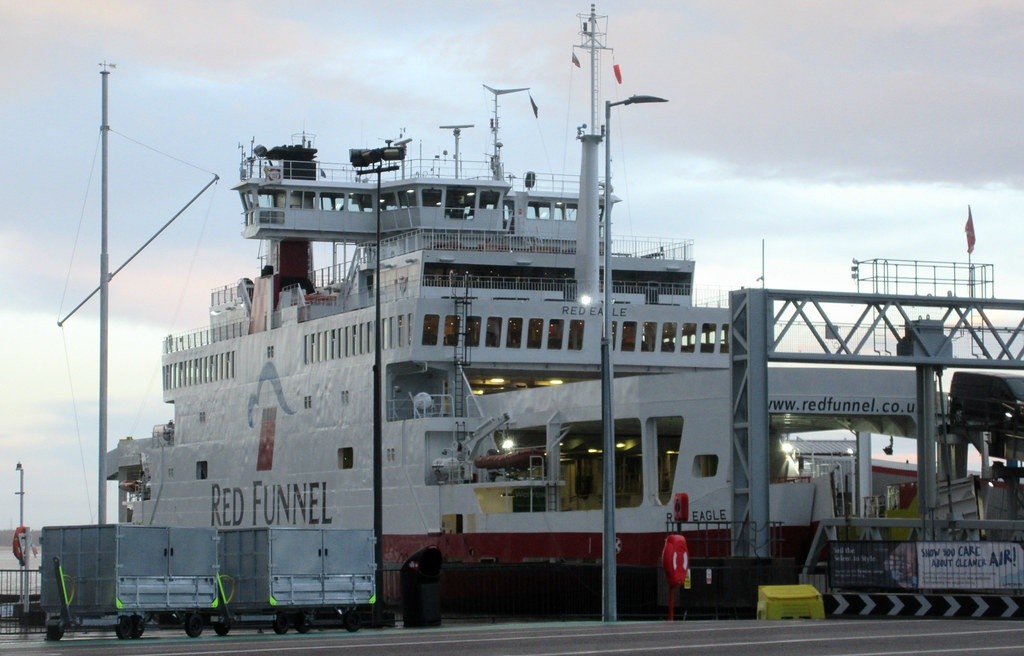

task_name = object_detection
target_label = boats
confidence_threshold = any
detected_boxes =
[104,86,953,627]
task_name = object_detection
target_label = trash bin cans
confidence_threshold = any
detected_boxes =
[400,544,445,627]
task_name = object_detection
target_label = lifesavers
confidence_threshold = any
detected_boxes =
[13,526,26,561]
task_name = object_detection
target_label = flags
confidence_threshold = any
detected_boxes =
[572,53,580,68]
[965,214,975,252]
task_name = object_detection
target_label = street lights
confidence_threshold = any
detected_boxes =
[13,462,25,601]
[348,139,405,618]
[602,93,674,625]
